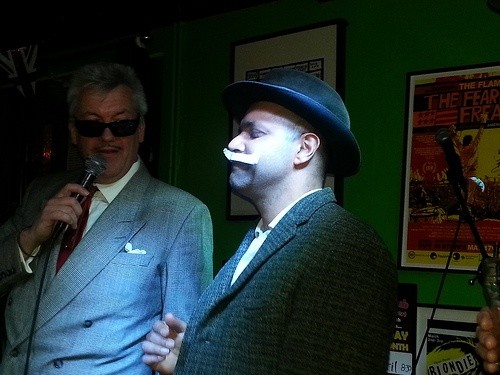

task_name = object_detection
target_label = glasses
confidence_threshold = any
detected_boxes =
[72,111,141,139]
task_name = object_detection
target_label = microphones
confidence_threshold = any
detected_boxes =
[51,152,108,243]
[435,128,469,193]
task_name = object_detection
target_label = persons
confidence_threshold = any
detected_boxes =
[451,114,487,220]
[0,63,213,375]
[476,309,500,372]
[142,69,399,375]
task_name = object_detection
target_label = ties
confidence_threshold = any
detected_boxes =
[55,182,100,277]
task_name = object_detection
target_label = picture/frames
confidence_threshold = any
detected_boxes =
[226,17,346,221]
[397,61,500,274]
[415,303,491,375]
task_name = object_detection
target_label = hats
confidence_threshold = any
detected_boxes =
[220,66,362,179]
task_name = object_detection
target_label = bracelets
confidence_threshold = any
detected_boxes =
[18,226,41,258]
